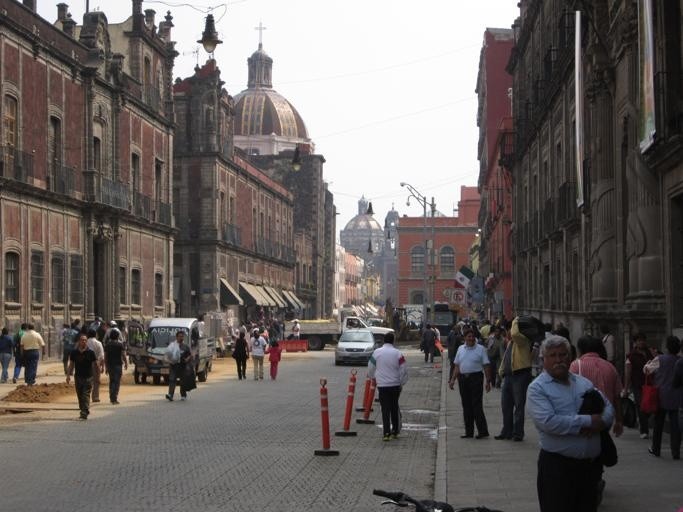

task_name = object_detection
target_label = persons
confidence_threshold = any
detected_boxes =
[0,327,14,383]
[365,332,410,440]
[59,315,135,405]
[267,340,280,379]
[22,322,45,383]
[64,334,97,420]
[230,311,284,349]
[11,322,28,383]
[600,324,618,366]
[525,330,616,510]
[249,330,267,379]
[569,334,625,504]
[234,332,249,380]
[419,315,575,441]
[624,331,654,439]
[292,320,300,342]
[163,331,191,401]
[636,335,680,462]
[674,339,682,406]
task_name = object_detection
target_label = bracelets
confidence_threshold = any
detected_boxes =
[615,420,623,427]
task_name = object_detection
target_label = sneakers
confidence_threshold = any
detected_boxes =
[165,394,173,401]
[78,410,92,420]
[460,431,524,441]
[110,400,121,405]
[181,397,186,401]
[382,431,399,442]
[640,433,649,440]
[238,375,277,382]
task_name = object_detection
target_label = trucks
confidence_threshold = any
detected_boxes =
[125,317,218,385]
[283,299,403,351]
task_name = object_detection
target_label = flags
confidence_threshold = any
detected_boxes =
[450,264,475,291]
[446,286,466,306]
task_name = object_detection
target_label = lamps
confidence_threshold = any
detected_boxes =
[197,13,225,53]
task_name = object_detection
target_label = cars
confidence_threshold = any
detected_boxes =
[332,329,377,367]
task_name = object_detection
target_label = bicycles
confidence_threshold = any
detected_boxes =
[369,486,503,512]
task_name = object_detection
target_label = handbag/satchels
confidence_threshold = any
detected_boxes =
[518,315,546,343]
[620,395,637,428]
[640,367,658,414]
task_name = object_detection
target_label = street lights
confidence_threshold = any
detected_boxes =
[398,181,438,356]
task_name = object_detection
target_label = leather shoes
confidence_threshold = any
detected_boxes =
[648,447,654,454]
